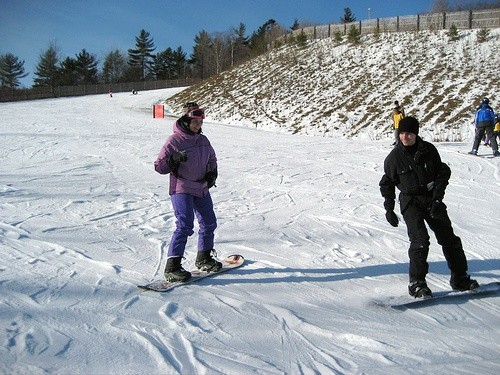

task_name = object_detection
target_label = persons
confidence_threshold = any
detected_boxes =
[378,116,480,299]
[153,102,222,282]
[393,107,405,145]
[109,88,112,93]
[483,137,490,146]
[109,90,112,97]
[132,87,135,94]
[467,96,500,158]
[393,100,405,145]
[494,115,499,139]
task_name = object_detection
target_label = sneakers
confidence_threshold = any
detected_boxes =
[450,272,480,291]
[493,149,500,156]
[195,250,222,271]
[408,280,433,299]
[468,149,477,155]
[163,256,192,281]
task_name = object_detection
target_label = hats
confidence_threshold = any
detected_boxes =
[482,98,490,103]
[181,101,199,116]
[398,116,419,134]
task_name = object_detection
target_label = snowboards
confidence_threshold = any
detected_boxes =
[371,282,500,307]
[456,150,500,157]
[137,255,244,292]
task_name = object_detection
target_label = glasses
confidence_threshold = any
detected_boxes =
[185,108,205,119]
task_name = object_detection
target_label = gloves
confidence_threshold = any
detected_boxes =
[171,150,188,162]
[385,210,399,227]
[427,198,440,218]
[202,174,217,188]
